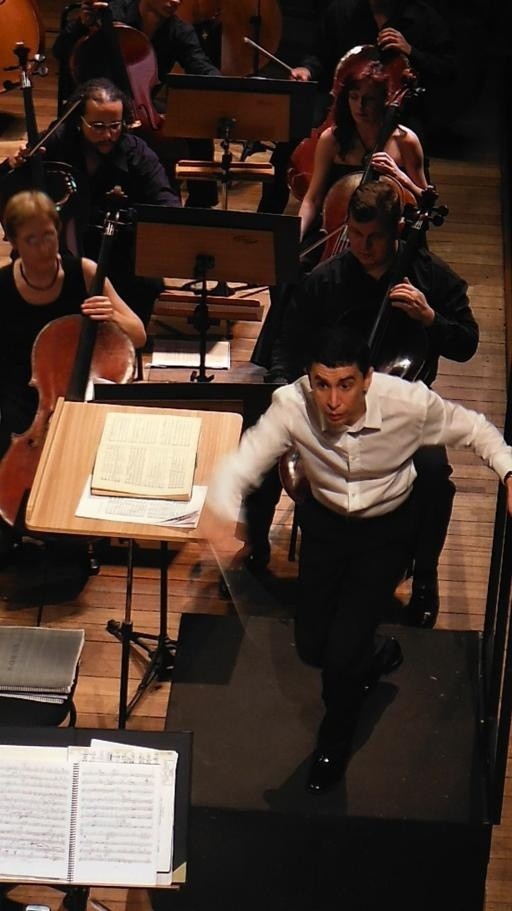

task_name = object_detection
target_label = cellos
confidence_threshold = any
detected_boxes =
[0,42,97,262]
[0,186,138,532]
[320,67,425,263]
[278,186,449,507]
[0,0,45,95]
[287,1,410,199]
[67,0,165,141]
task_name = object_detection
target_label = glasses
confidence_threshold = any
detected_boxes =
[80,115,123,133]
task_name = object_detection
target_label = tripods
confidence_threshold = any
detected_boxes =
[107,541,179,720]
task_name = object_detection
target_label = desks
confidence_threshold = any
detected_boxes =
[26,395,245,729]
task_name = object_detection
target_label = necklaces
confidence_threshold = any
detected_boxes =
[18,255,61,291]
[353,124,383,166]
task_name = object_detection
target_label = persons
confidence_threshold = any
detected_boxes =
[1,191,148,573]
[253,1,457,215]
[182,329,511,793]
[0,80,182,336]
[292,60,433,263]
[247,179,482,629]
[51,0,226,208]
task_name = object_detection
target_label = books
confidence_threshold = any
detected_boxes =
[172,158,277,184]
[91,411,203,503]
[151,275,270,324]
[1,739,179,886]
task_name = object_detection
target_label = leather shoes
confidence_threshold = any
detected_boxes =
[405,568,442,630]
[302,637,405,796]
